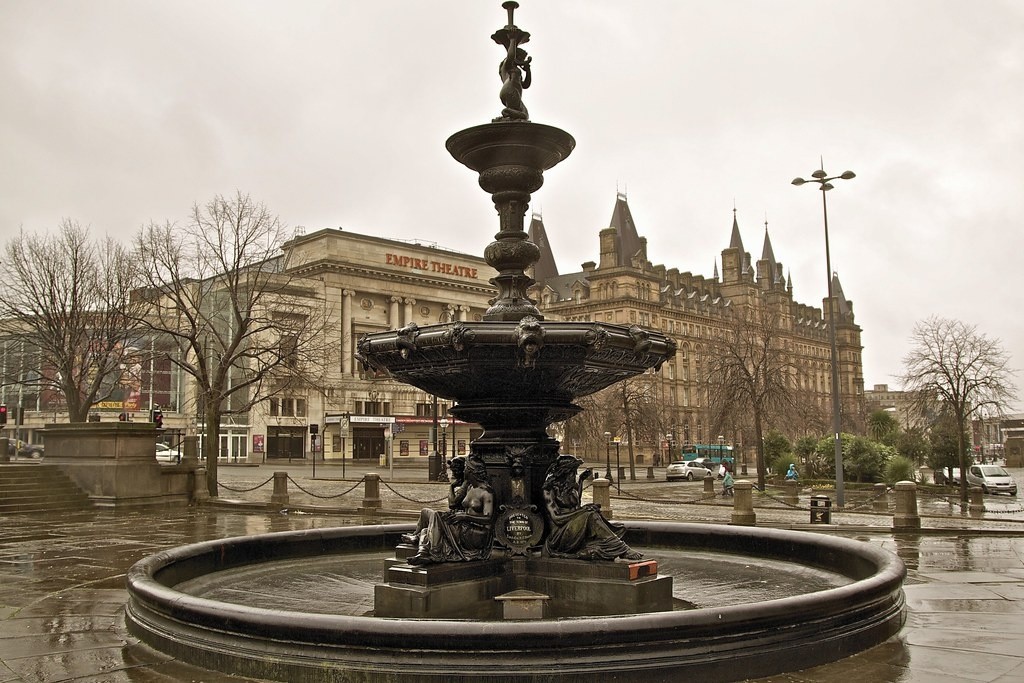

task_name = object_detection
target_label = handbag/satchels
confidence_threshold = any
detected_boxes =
[718,464,726,476]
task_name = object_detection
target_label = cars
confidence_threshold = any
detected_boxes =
[155,442,184,466]
[968,464,1018,496]
[0,436,44,460]
[933,464,969,486]
[666,460,712,482]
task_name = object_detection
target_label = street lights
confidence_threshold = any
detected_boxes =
[790,169,857,513]
[665,433,673,464]
[979,441,986,465]
[437,414,450,482]
[603,431,614,486]
[717,435,725,479]
[761,436,768,476]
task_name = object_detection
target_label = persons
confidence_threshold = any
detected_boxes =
[492,27,531,122]
[407,456,494,566]
[722,461,733,496]
[787,464,799,479]
[401,457,469,545]
[653,453,660,466]
[542,455,643,560]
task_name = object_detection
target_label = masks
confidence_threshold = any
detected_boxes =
[792,467,795,470]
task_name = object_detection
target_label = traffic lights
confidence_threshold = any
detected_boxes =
[119,413,126,421]
[151,409,163,428]
[0,403,7,424]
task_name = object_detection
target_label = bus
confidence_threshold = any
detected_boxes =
[679,443,737,476]
